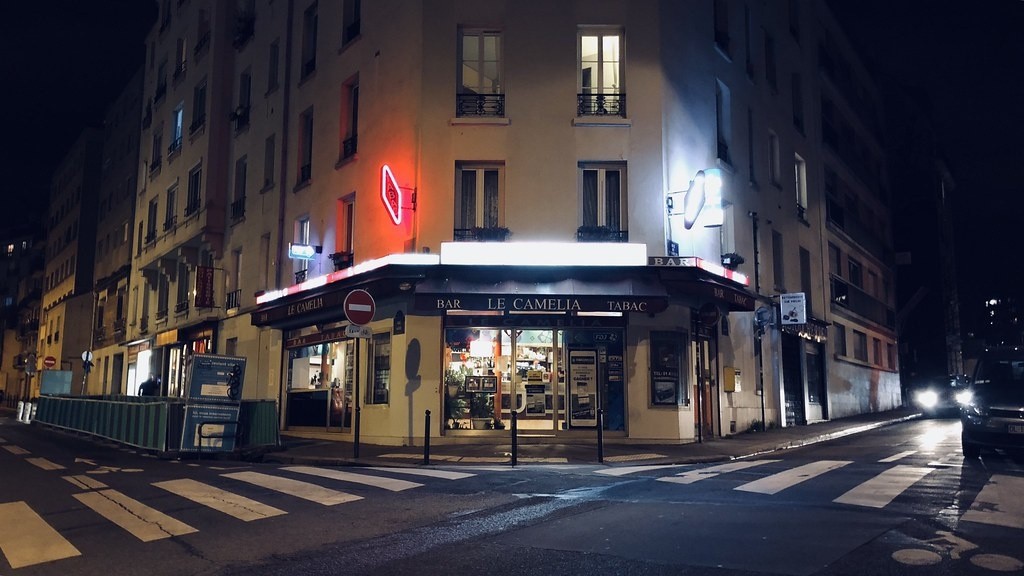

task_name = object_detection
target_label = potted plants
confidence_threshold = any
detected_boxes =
[469,394,496,430]
[493,415,506,431]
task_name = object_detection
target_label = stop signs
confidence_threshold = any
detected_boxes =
[344,290,376,326]
[44,355,56,368]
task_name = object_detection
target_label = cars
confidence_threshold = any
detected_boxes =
[961,353,1024,459]
[923,375,968,419]
